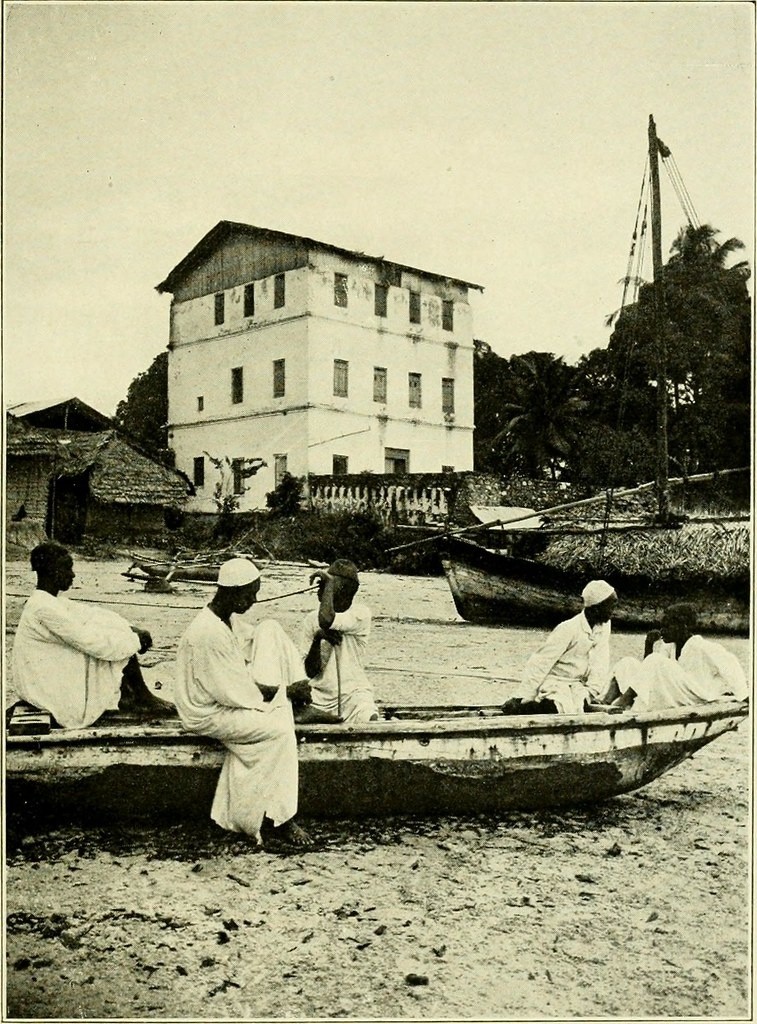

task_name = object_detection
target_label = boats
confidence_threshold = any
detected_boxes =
[136,561,267,580]
[6,696,749,818]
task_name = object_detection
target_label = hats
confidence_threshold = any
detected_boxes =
[580,579,615,608]
[215,558,262,588]
[326,559,359,583]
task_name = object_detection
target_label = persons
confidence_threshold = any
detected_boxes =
[300,559,379,723]
[12,543,177,729]
[601,604,749,714]
[501,580,618,715]
[177,558,344,847]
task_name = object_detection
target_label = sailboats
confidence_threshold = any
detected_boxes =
[439,112,753,636]
